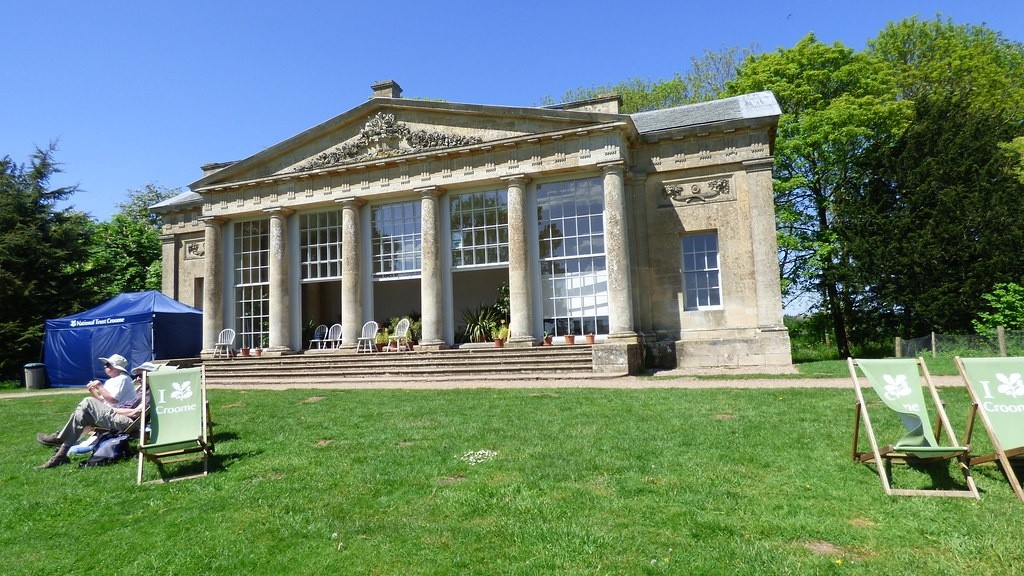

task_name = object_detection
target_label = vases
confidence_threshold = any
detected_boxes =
[564,335,575,345]
[585,335,594,345]
[255,349,262,357]
[242,348,250,357]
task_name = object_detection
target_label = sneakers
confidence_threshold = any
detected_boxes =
[33,455,71,471]
[36,431,63,447]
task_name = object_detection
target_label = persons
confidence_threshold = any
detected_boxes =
[53,352,137,452]
[33,353,158,472]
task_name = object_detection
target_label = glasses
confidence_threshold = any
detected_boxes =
[133,374,141,378]
[103,362,110,367]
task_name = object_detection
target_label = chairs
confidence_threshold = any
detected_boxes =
[137,365,215,486]
[847,357,981,501]
[953,356,1024,504]
[308,325,328,349]
[356,321,378,353]
[213,328,236,358]
[323,324,343,349]
[386,319,411,352]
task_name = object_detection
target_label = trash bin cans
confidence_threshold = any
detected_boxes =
[23,362,45,391]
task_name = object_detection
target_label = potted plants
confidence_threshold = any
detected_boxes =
[491,324,508,347]
[374,314,414,350]
[542,330,553,346]
[457,281,510,342]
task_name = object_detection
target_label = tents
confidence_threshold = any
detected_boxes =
[45,288,204,388]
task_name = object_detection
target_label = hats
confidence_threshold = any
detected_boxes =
[130,362,162,374]
[98,353,128,373]
